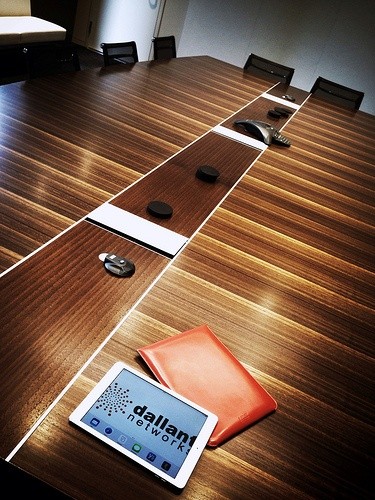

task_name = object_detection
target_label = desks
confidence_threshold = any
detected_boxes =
[0,55,375,500]
[0,16,67,45]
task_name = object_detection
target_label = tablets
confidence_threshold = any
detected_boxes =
[69,361,218,491]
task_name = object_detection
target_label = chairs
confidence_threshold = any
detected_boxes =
[310,76,365,109]
[153,35,176,60]
[101,41,137,66]
[28,44,80,79]
[244,53,295,86]
